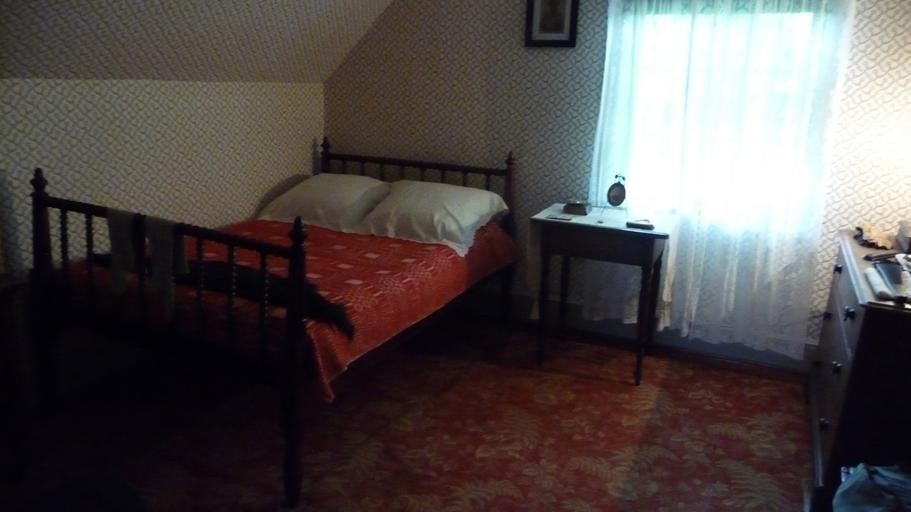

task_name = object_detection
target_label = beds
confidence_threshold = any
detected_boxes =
[29,136,517,509]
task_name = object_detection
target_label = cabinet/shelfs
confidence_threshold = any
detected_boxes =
[805,230,911,512]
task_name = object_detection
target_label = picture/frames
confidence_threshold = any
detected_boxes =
[524,0,579,47]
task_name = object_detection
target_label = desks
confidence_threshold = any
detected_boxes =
[529,203,679,386]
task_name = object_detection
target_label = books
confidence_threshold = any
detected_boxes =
[875,259,910,302]
[561,198,593,216]
[862,268,895,300]
[543,212,575,221]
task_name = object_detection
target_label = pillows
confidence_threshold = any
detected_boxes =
[256,173,509,257]
